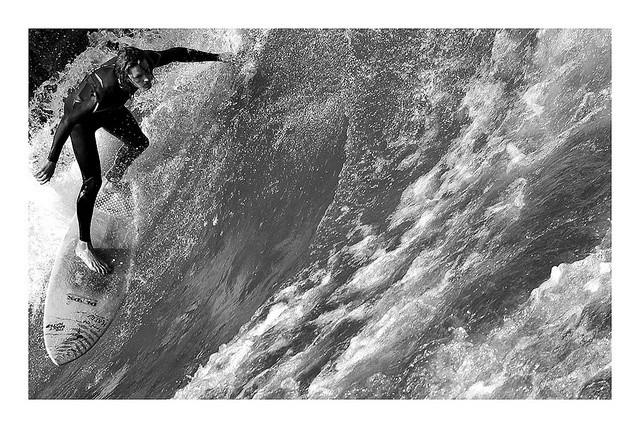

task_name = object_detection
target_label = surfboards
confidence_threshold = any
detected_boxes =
[42,180,139,368]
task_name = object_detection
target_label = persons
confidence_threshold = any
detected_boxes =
[34,40,237,275]
